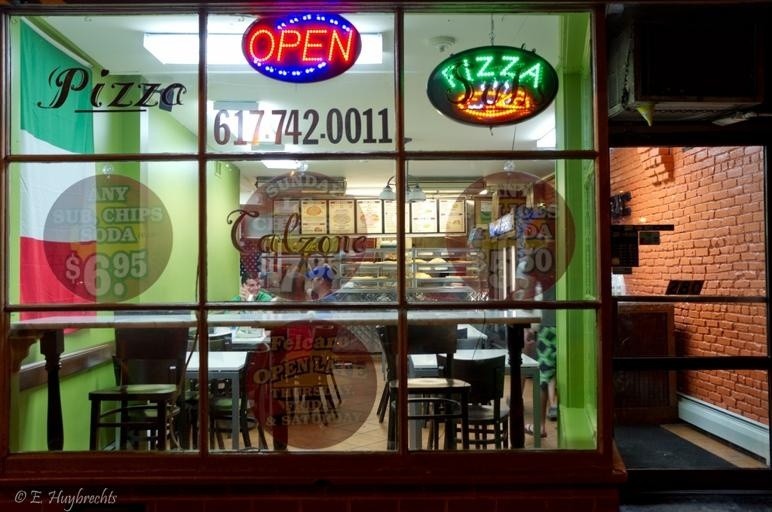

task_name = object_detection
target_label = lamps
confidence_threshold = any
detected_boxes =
[380,174,427,203]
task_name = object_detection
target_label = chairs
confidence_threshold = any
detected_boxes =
[86,330,190,453]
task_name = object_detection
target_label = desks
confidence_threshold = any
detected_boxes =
[114,321,542,451]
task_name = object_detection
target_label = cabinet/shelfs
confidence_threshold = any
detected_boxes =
[329,247,483,294]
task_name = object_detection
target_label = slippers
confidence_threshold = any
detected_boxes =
[527,422,549,437]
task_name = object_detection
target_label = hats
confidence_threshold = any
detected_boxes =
[304,265,334,279]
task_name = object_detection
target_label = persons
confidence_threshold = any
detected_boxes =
[224,263,338,314]
[521,320,558,438]
